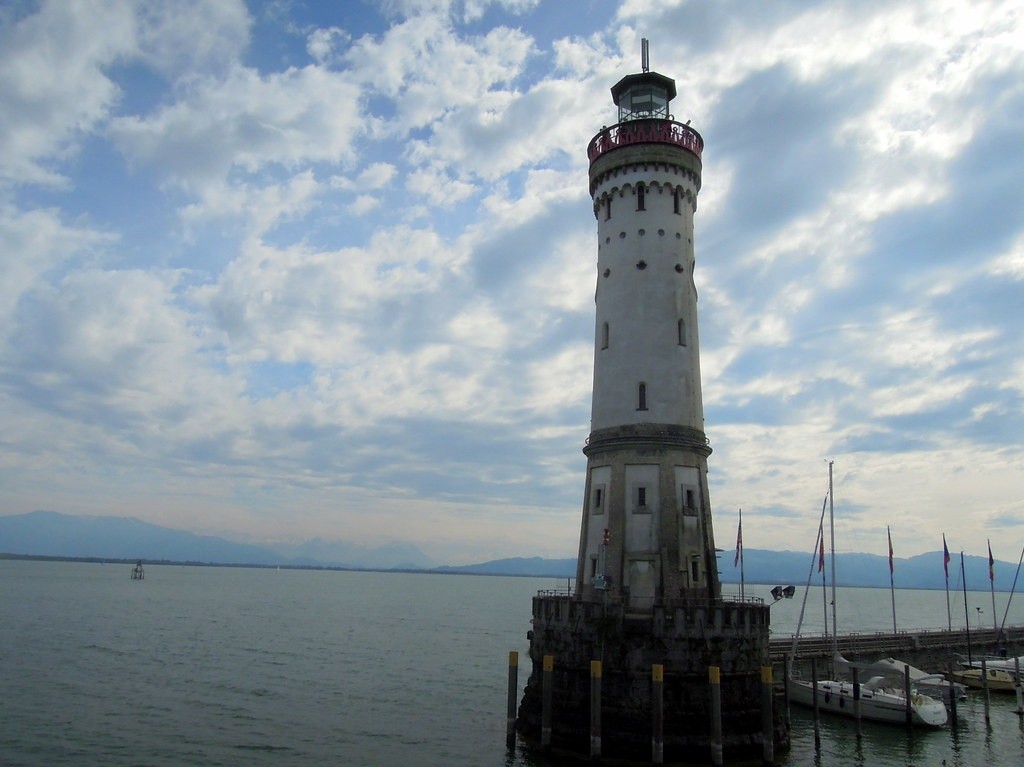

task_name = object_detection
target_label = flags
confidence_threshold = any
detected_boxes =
[888,533,894,571]
[817,533,824,572]
[733,520,742,567]
[989,547,994,582]
[944,539,950,577]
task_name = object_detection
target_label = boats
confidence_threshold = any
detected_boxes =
[785,460,1024,725]
